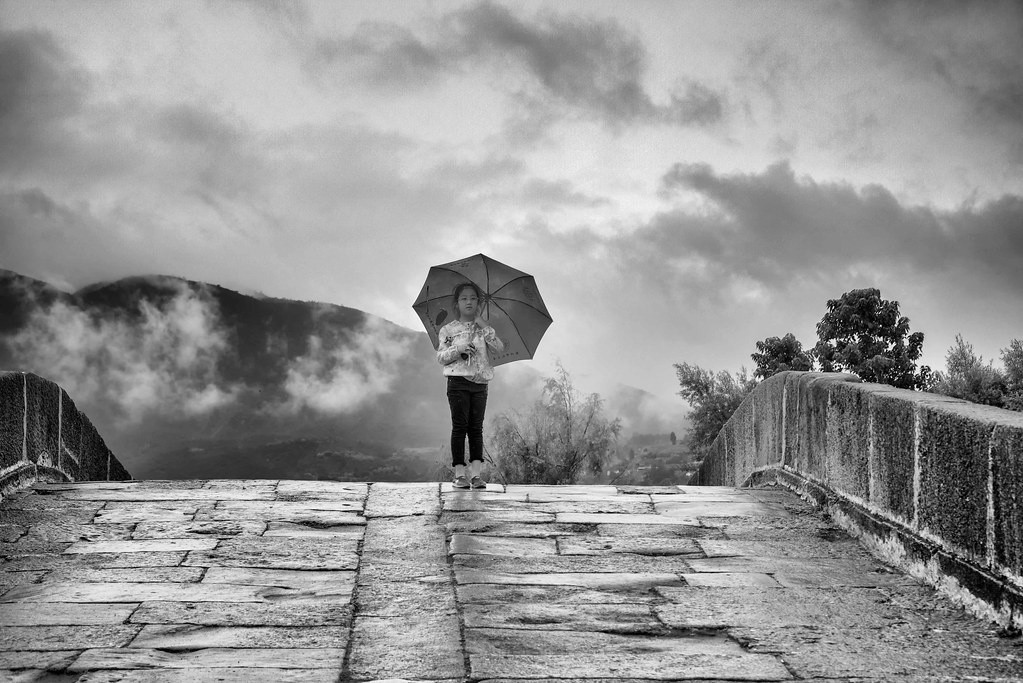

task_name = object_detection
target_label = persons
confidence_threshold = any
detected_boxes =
[437,284,505,489]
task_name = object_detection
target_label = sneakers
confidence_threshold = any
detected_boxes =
[452,476,470,488]
[471,476,487,488]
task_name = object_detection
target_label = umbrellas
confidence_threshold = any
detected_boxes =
[411,253,554,366]
